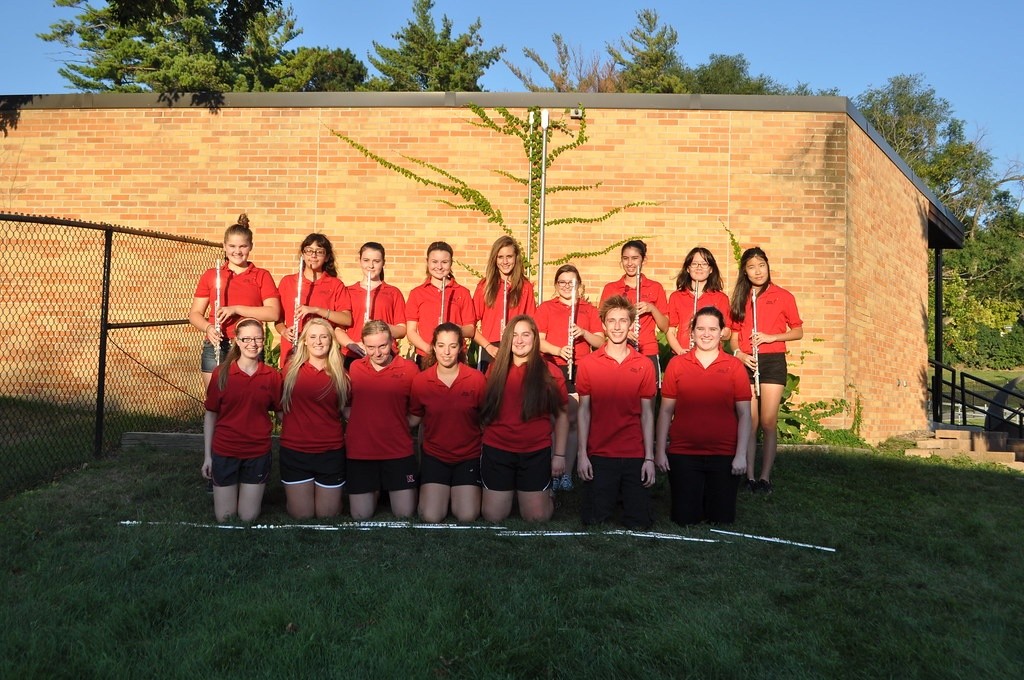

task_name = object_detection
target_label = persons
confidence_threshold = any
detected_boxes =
[334,242,407,423]
[598,240,670,389]
[189,224,282,400]
[732,247,803,495]
[344,320,421,521]
[467,236,535,375]
[481,315,570,524]
[404,241,477,372]
[658,307,752,524]
[406,322,489,524]
[536,263,605,492]
[200,317,284,523]
[274,233,352,421]
[575,296,657,529]
[666,247,732,356]
[282,319,351,520]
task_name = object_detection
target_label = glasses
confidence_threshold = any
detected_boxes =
[302,246,326,256]
[557,280,578,287]
[690,261,710,269]
[237,337,264,343]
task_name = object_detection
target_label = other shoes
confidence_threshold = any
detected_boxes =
[552,474,574,493]
[743,477,772,495]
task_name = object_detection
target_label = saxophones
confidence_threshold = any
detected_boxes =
[499,280,507,342]
[689,280,698,350]
[752,289,760,396]
[364,272,371,325]
[439,276,445,326]
[215,258,221,366]
[567,278,575,380]
[634,266,640,352]
[294,257,303,346]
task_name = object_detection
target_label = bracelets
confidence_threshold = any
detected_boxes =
[484,342,493,352]
[554,453,568,459]
[645,457,656,464]
[325,309,331,319]
[734,348,741,357]
